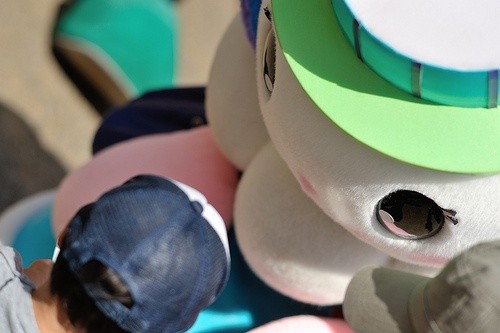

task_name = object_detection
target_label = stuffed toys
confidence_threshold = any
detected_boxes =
[1,0,500,333]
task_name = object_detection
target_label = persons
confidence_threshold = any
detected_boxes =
[0,171,232,333]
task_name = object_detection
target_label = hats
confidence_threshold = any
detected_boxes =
[342,239,499,332]
[61,173,229,333]
